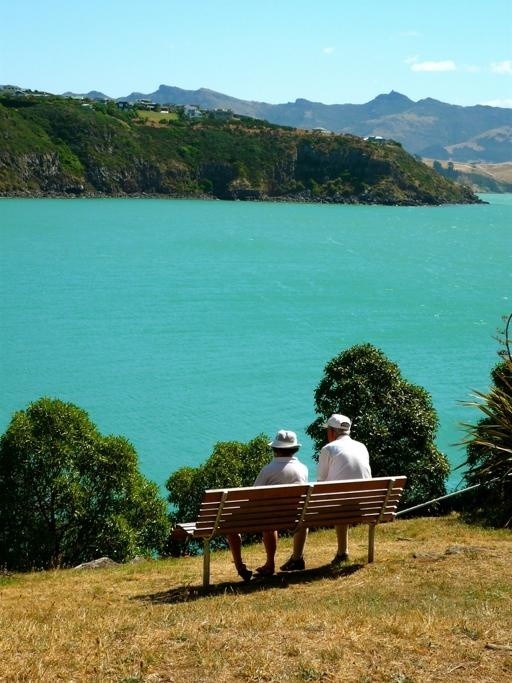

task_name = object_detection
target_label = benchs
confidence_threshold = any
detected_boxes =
[175,475,407,589]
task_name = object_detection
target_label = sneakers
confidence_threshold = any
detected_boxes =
[280,559,305,571]
[257,566,274,576]
[331,553,347,565]
[240,568,252,579]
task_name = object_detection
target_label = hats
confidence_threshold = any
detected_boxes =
[322,414,352,430]
[268,430,302,449]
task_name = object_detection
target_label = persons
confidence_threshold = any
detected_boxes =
[280,414,373,571]
[226,429,308,581]
